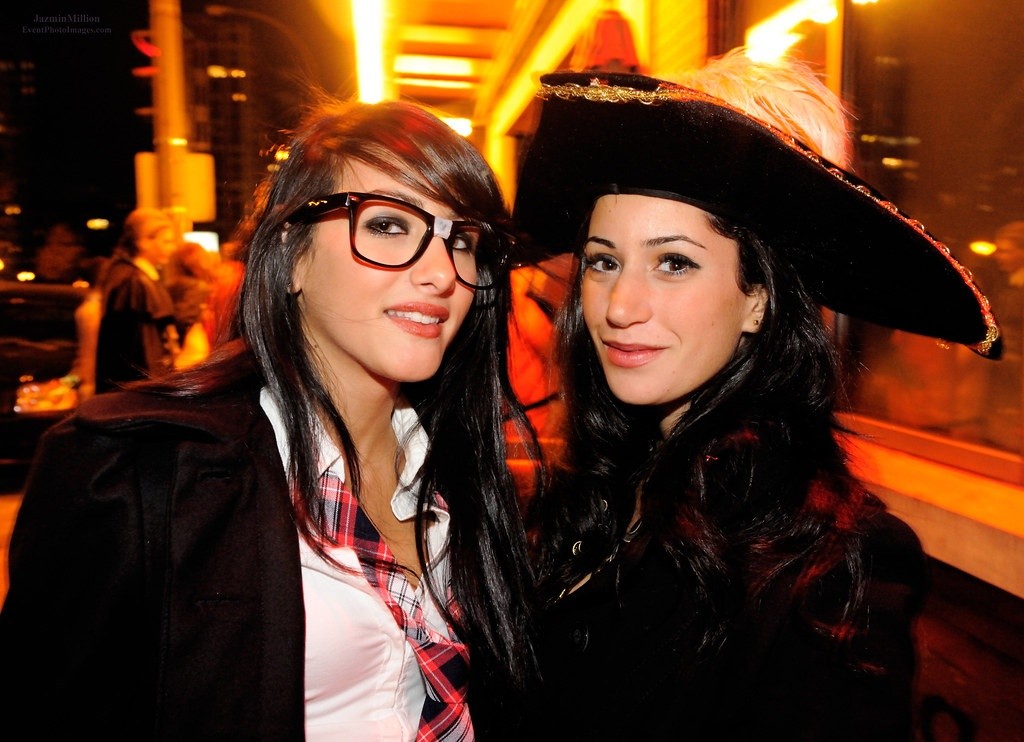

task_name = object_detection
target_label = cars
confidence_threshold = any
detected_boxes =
[0,278,92,497]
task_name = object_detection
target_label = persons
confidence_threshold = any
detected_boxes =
[507,55,1006,742]
[994,220,1024,289]
[0,101,547,742]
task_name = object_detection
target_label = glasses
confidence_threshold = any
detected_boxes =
[282,191,504,289]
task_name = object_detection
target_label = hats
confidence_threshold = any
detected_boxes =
[538,40,1011,358]
